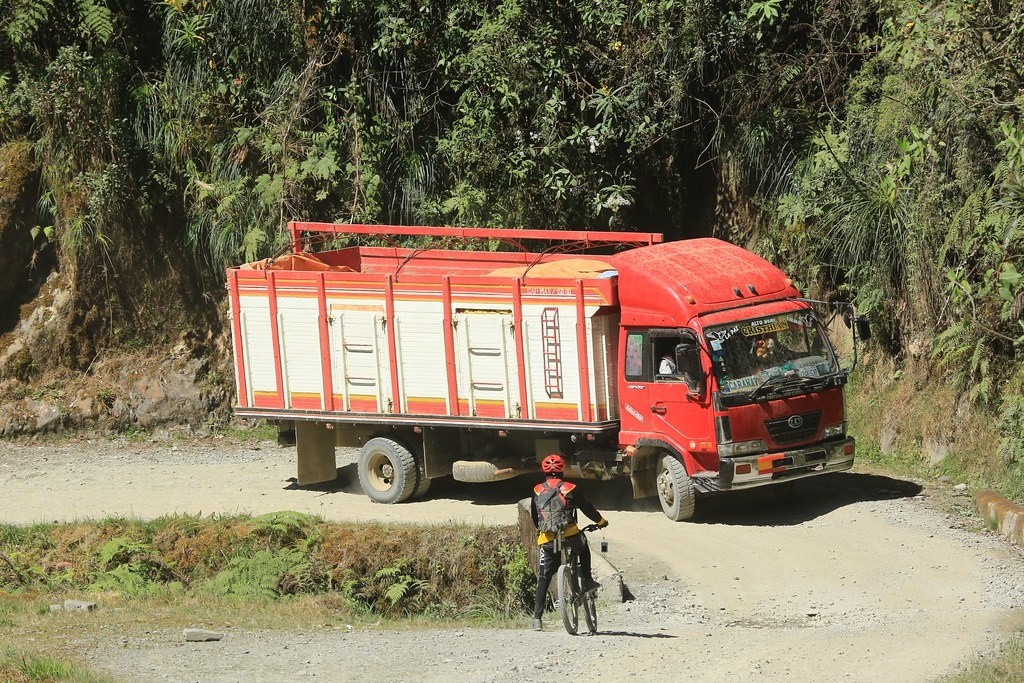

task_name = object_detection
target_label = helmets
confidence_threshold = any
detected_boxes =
[542,454,565,473]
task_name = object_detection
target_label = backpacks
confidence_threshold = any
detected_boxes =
[538,481,573,532]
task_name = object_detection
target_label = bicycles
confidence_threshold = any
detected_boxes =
[552,513,609,637]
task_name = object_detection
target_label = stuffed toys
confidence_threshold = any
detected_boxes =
[757,339,778,359]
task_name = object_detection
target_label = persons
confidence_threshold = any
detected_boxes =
[659,340,683,380]
[530,454,608,631]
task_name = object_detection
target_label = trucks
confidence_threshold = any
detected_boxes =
[224,219,859,520]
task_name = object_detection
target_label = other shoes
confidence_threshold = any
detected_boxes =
[534,619,543,631]
[585,579,600,591]
